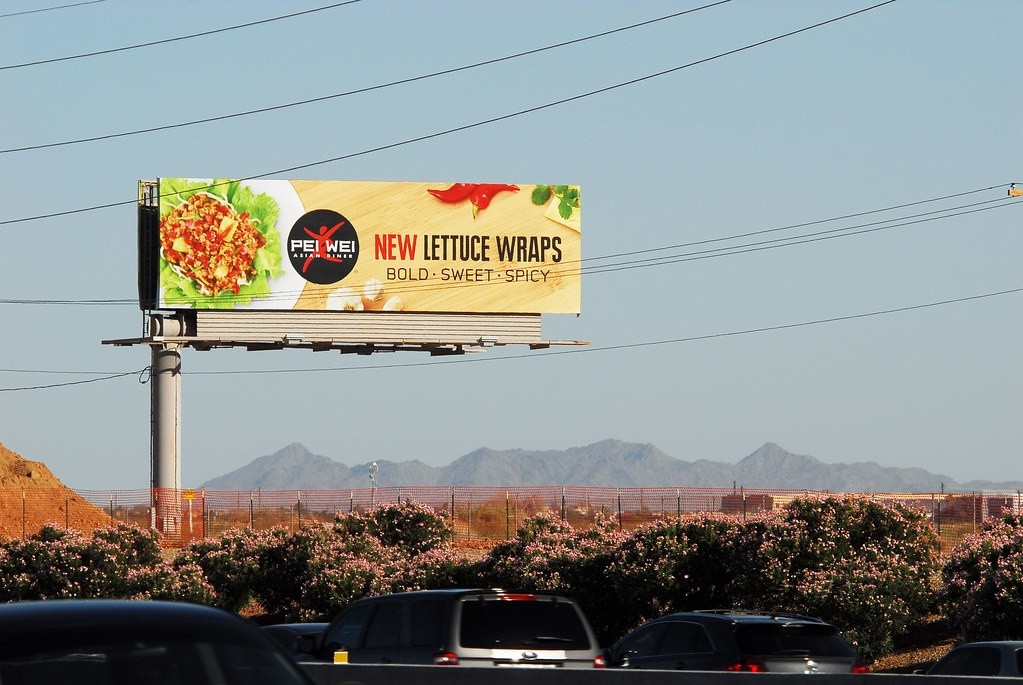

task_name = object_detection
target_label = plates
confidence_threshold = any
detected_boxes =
[160,179,307,310]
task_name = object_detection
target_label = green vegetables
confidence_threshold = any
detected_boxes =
[531,185,580,220]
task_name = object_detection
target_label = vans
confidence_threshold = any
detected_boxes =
[292,587,608,669]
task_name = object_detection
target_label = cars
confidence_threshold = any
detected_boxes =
[4,618,359,666]
[912,638,1022,678]
[0,598,314,685]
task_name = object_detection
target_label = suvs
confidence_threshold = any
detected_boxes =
[602,607,872,674]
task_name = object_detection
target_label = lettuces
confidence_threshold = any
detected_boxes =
[159,178,286,309]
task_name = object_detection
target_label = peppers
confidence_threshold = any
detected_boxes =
[427,182,521,219]
